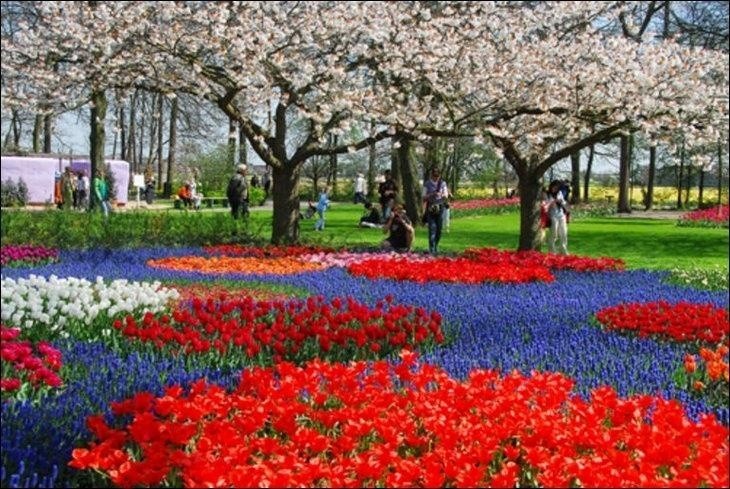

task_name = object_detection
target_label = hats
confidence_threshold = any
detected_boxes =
[431,167,440,175]
[395,205,406,212]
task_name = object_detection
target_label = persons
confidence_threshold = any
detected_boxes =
[381,204,415,251]
[55,167,113,217]
[352,171,369,205]
[261,170,272,192]
[378,170,399,224]
[188,178,202,211]
[421,165,449,254]
[314,186,332,231]
[226,163,248,219]
[249,172,261,187]
[541,179,579,256]
[298,199,317,219]
[358,203,385,229]
[178,184,192,211]
